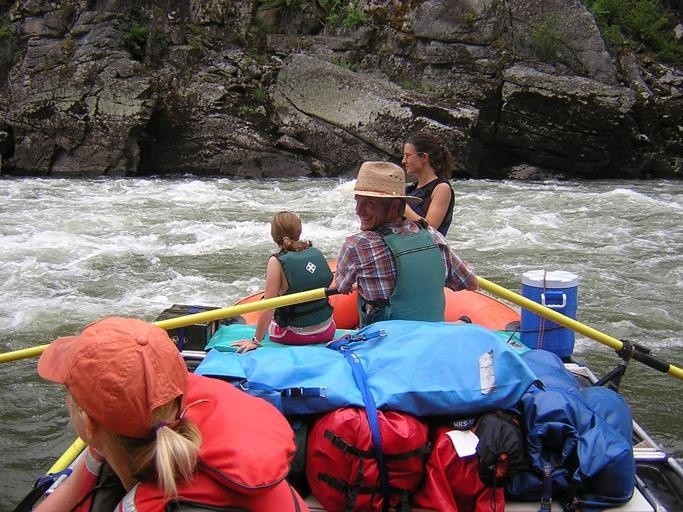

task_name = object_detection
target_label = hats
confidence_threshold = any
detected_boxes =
[38,317,191,440]
[336,161,423,203]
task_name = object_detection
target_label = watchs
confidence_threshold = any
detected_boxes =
[251,337,261,345]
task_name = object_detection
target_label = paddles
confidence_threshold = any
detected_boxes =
[10,439,85,512]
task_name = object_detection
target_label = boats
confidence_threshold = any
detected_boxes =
[8,255,683,512]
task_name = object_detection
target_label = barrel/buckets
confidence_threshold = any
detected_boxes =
[521,271,579,359]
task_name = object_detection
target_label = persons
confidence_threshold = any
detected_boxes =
[230,211,336,354]
[401,133,454,237]
[341,161,479,329]
[32,316,308,512]
